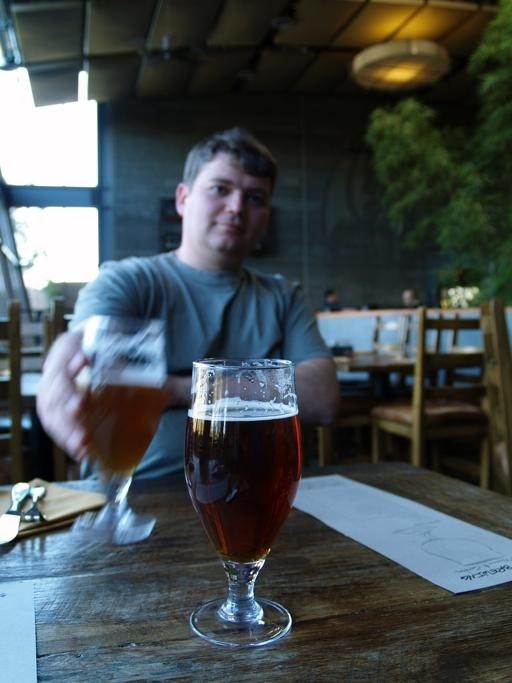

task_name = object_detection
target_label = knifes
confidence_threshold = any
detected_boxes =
[0,482,30,545]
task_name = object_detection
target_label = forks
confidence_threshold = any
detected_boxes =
[20,486,47,522]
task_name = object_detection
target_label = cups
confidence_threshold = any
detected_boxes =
[185,358,302,649]
[71,314,167,547]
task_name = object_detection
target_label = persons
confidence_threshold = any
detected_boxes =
[34,125,339,510]
[317,284,343,315]
[395,287,424,310]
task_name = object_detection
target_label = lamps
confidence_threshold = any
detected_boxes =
[349,39,452,91]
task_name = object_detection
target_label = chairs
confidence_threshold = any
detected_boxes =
[309,297,512,487]
[44,298,80,482]
[0,299,22,485]
